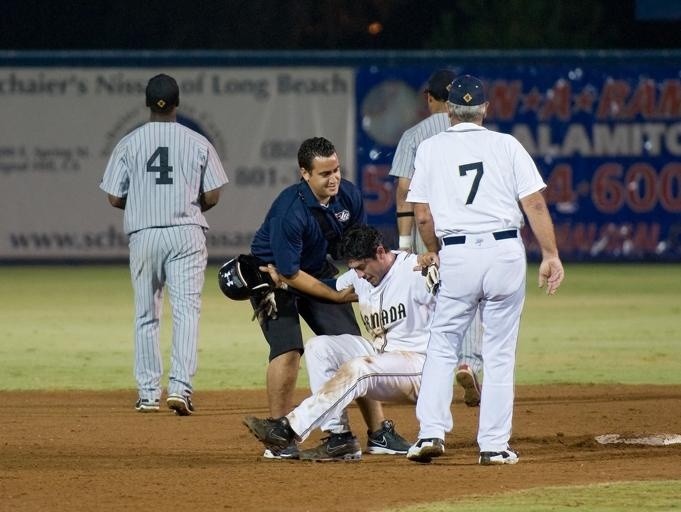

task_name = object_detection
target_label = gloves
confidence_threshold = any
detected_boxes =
[251,291,278,321]
[424,262,441,296]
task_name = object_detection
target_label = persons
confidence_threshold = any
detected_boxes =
[406,74,566,465]
[251,138,412,459]
[389,71,485,406]
[243,224,440,461]
[99,74,230,415]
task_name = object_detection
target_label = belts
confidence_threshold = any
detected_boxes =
[443,230,517,245]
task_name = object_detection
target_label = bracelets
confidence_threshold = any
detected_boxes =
[399,235,412,248]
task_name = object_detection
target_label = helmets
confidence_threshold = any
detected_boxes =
[218,254,275,300]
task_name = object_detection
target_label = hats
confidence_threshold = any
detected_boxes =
[424,69,456,99]
[448,75,485,106]
[146,74,179,113]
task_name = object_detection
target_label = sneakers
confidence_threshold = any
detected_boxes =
[166,393,195,416]
[456,365,481,407]
[242,417,522,463]
[135,398,160,413]
